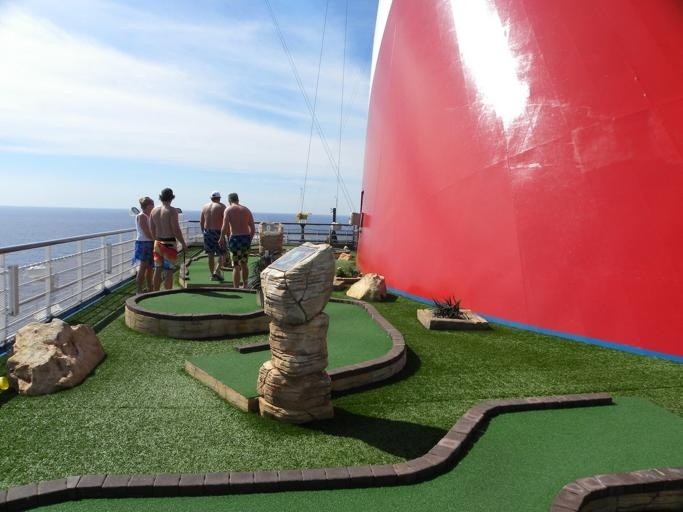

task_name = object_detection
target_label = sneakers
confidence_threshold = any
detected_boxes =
[210,270,224,281]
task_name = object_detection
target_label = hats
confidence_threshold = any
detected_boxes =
[211,192,221,198]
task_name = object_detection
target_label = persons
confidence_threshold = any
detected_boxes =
[149,187,187,291]
[134,196,154,293]
[198,192,230,280]
[218,193,255,289]
